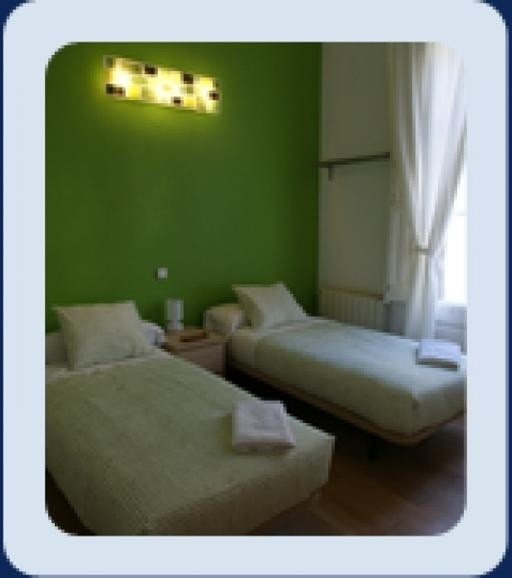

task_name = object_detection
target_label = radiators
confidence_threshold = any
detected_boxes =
[316,288,384,331]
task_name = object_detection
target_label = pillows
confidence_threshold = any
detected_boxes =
[58,303,157,371]
[230,282,311,333]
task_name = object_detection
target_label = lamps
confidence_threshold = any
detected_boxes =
[165,299,184,331]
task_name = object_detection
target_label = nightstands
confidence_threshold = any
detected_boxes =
[165,326,229,374]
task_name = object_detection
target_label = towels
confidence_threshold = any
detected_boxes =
[230,400,294,453]
[416,339,461,370]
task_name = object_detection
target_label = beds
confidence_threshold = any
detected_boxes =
[201,282,466,447]
[45,301,336,535]
